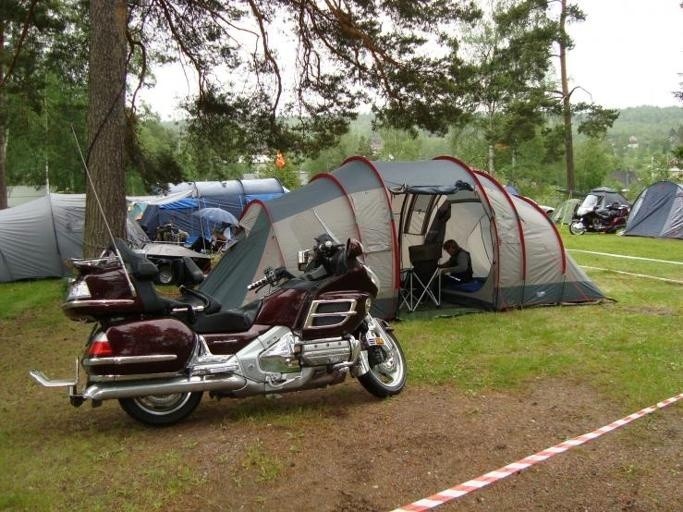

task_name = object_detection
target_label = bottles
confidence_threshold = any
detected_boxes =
[155,223,179,243]
[210,242,213,255]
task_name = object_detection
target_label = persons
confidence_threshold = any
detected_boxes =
[417,239,473,305]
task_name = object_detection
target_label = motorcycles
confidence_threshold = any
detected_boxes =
[30,124,407,427]
[568,195,630,236]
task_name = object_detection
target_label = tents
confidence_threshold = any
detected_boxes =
[546,198,583,225]
[621,180,683,238]
[194,153,616,324]
[0,175,291,286]
[573,187,631,229]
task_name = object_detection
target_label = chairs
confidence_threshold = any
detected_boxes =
[217,240,228,254]
[404,242,443,313]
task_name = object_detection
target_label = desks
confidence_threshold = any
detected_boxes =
[399,268,413,312]
